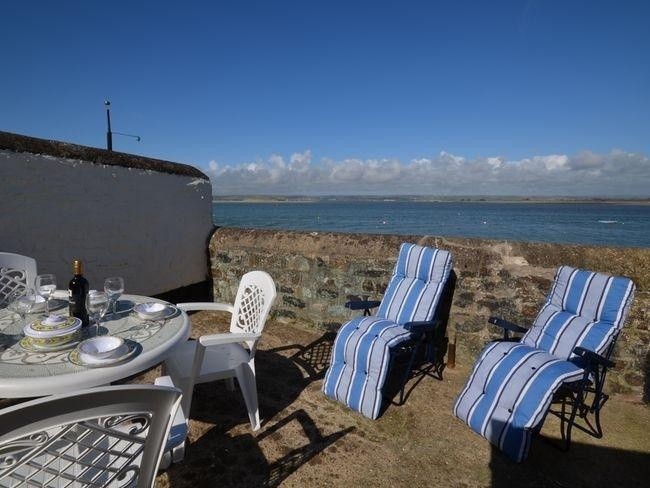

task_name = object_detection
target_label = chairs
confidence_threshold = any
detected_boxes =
[162,270,278,433]
[321,240,454,420]
[0,252,40,309]
[453,262,637,462]
[0,383,183,488]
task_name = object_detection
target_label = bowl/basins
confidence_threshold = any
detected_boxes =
[27,296,46,310]
[24,317,81,348]
[76,335,125,361]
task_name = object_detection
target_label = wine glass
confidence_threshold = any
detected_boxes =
[13,288,34,324]
[105,276,125,319]
[87,292,110,336]
[34,273,58,316]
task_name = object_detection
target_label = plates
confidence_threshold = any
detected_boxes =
[16,334,82,352]
[131,301,180,322]
[11,302,64,312]
[67,340,139,367]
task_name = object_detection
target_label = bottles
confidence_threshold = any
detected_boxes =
[68,260,90,329]
